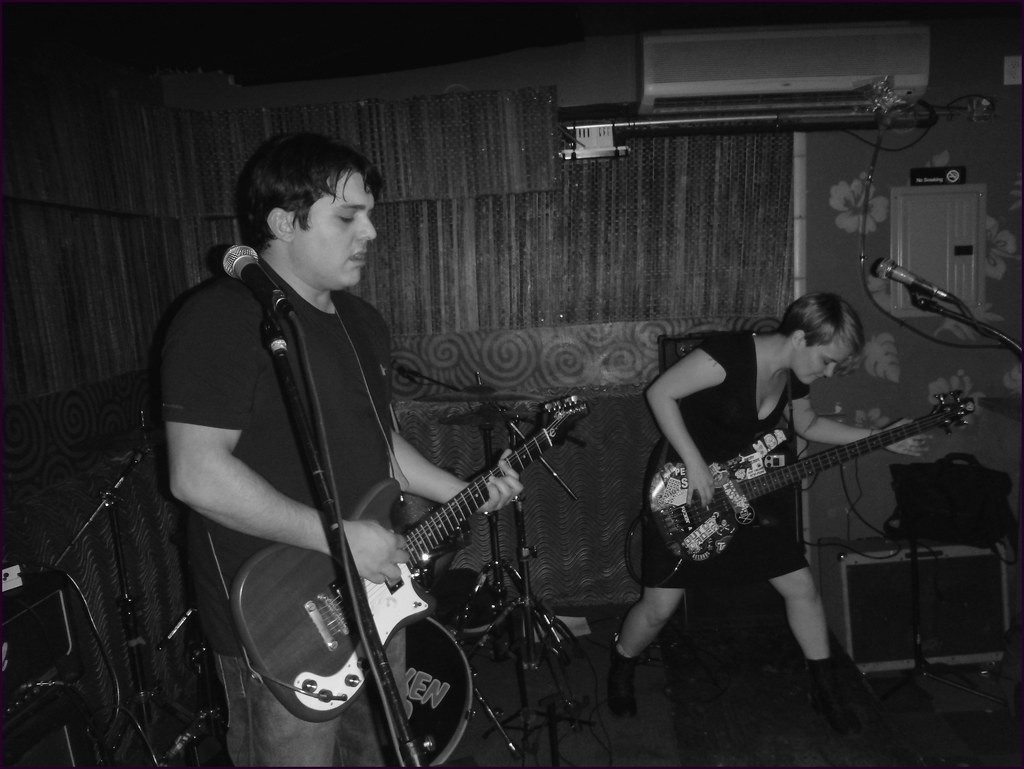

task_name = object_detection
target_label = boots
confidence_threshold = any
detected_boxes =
[804,653,862,738]
[607,633,638,720]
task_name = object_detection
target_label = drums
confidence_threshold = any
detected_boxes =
[391,468,472,566]
[367,616,473,768]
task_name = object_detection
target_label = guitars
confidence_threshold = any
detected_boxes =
[648,390,974,560]
[232,394,588,722]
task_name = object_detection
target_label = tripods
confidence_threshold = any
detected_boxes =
[876,527,1011,713]
[50,446,232,766]
[407,369,598,742]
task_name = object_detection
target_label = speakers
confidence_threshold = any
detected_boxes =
[817,534,1009,673]
[658,335,803,633]
[3,687,97,767]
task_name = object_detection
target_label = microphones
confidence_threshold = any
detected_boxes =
[392,359,417,383]
[872,258,957,304]
[223,244,294,314]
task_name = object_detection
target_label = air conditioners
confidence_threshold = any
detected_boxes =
[639,22,931,117]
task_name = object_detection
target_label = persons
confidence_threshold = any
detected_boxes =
[609,292,933,735]
[157,130,525,769]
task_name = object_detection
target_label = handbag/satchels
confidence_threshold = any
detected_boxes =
[888,452,1015,550]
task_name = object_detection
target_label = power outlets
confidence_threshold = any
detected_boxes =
[1003,55,1022,86]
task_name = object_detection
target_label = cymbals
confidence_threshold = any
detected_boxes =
[67,425,168,452]
[439,412,534,426]
[411,387,538,402]
